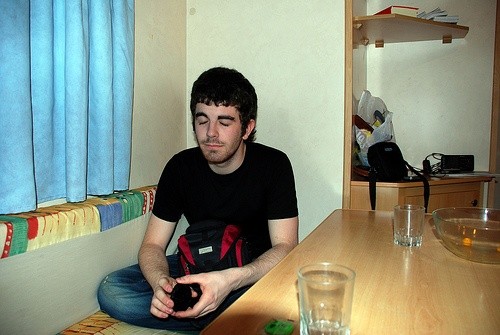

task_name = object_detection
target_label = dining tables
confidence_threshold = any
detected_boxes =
[198,209,499,335]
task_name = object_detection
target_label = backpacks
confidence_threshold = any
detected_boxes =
[178,219,265,276]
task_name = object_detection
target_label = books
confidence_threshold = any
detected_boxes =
[374,6,459,24]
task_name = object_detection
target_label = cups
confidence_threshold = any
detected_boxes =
[394,204,425,247]
[298,262,355,335]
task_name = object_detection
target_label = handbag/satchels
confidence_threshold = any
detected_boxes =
[355,112,396,167]
[367,142,408,183]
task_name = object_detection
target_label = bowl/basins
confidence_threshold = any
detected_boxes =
[432,208,500,265]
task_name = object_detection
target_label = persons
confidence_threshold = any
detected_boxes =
[97,67,298,330]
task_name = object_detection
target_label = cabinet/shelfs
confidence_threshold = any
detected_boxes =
[350,174,492,211]
